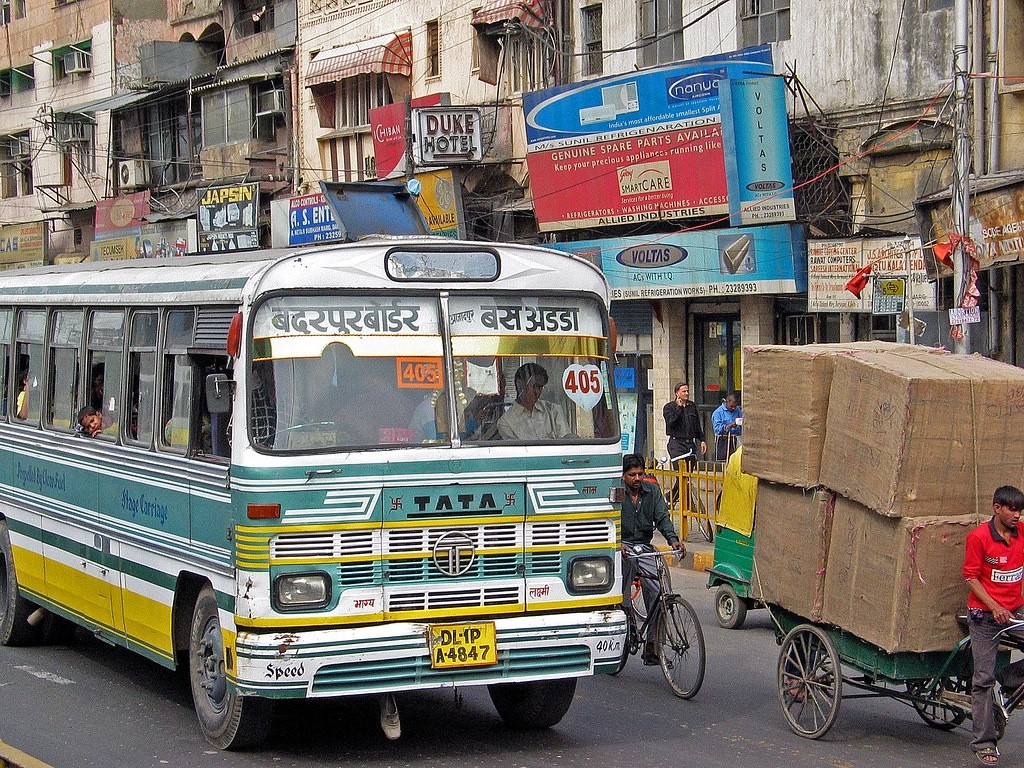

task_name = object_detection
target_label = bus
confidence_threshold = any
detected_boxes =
[0,234,627,751]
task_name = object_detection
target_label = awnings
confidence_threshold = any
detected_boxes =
[30,36,94,64]
[304,33,411,127]
[26,89,163,124]
[0,127,30,145]
[471,0,551,32]
[0,62,35,86]
[36,202,97,213]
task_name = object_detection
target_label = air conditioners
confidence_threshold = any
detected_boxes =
[119,160,149,187]
[59,121,91,143]
[10,136,29,157]
[63,51,90,74]
[256,90,285,117]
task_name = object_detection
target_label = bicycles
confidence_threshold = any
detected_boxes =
[604,545,706,700]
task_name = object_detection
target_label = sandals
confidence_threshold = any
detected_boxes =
[975,747,999,766]
[1007,692,1024,711]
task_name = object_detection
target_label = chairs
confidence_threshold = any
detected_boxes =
[481,402,513,440]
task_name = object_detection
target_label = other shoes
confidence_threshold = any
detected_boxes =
[643,652,669,665]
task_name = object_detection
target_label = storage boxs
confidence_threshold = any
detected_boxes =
[740,338,1024,656]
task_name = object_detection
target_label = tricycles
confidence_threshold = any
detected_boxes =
[704,444,1023,739]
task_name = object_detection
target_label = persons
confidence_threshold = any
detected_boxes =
[623,453,687,664]
[16,369,41,419]
[663,382,707,505]
[711,393,742,486]
[92,363,487,453]
[961,485,1024,765]
[75,407,119,436]
[497,363,577,440]
